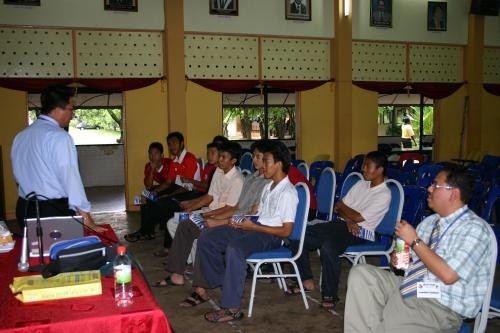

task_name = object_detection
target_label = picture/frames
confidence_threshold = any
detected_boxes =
[427,2,447,31]
[3,0,41,6]
[285,0,311,21]
[104,0,138,12]
[370,0,392,28]
[209,0,238,16]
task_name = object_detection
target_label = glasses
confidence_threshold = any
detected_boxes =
[432,182,454,191]
[64,108,75,114]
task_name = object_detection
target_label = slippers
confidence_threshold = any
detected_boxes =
[150,276,185,288]
[178,292,211,308]
[285,283,311,296]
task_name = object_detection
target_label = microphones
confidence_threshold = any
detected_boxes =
[18,222,29,272]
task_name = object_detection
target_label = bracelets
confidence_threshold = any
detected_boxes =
[84,217,90,220]
[411,238,422,249]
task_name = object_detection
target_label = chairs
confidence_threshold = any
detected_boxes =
[240,153,500,332]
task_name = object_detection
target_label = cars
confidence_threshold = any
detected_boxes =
[77,122,100,130]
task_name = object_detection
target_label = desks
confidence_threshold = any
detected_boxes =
[0,224,174,332]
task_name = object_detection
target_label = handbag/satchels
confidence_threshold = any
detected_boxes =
[42,235,110,280]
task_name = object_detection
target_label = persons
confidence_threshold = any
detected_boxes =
[124,132,201,243]
[144,142,172,190]
[286,151,391,305]
[402,117,417,148]
[285,157,317,222]
[150,137,270,307]
[344,164,497,333]
[205,142,299,321]
[10,81,108,232]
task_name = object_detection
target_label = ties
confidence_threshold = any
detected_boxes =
[399,219,439,299]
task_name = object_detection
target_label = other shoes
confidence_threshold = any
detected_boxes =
[204,308,244,323]
[154,247,170,258]
[124,231,143,243]
[139,232,156,240]
[320,295,340,310]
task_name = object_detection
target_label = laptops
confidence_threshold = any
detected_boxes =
[24,216,83,257]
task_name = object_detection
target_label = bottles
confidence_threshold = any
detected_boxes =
[113,246,133,306]
[392,235,410,277]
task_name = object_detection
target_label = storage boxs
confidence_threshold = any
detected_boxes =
[10,269,102,302]
